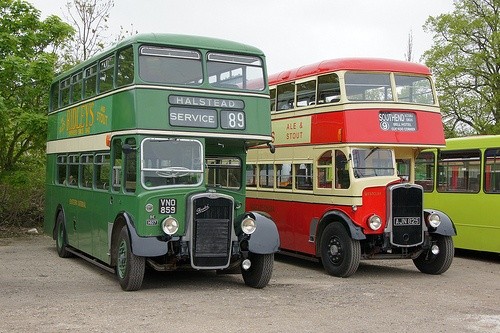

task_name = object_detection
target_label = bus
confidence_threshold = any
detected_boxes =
[326,135,500,253]
[42,32,278,292]
[207,55,458,279]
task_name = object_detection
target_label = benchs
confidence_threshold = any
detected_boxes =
[414,171,500,193]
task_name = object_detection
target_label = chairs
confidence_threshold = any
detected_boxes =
[287,93,327,109]
[113,165,137,189]
[296,169,313,190]
